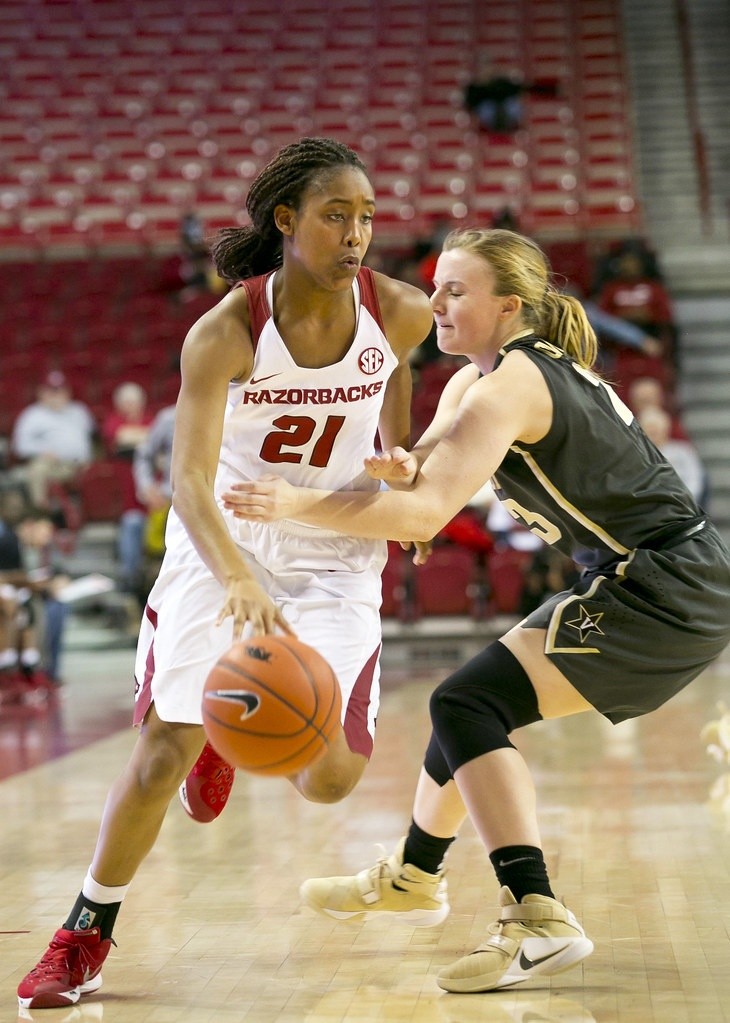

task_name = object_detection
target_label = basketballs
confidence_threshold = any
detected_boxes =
[200,633,345,782]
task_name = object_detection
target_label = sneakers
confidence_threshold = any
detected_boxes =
[178,740,236,824]
[300,837,450,931]
[437,886,593,993]
[16,926,112,1009]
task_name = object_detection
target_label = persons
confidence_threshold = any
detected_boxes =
[2,241,708,683]
[219,229,730,992]
[17,137,435,1009]
